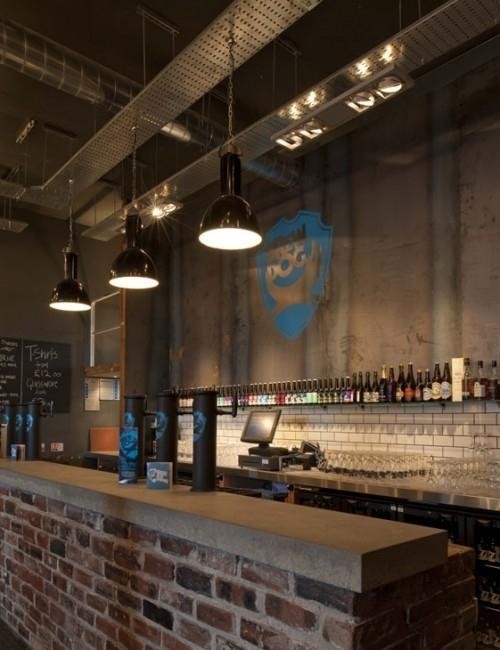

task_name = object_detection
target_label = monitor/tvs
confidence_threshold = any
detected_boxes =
[241,409,288,456]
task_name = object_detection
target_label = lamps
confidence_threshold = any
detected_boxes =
[48,178,94,312]
[109,119,160,292]
[199,1,265,252]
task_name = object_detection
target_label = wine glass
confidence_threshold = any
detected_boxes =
[424,432,499,489]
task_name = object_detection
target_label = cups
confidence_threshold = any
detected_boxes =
[318,446,434,484]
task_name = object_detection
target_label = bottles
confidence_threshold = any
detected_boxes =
[480,626,499,646]
[171,359,499,409]
[478,574,499,608]
[477,531,499,566]
